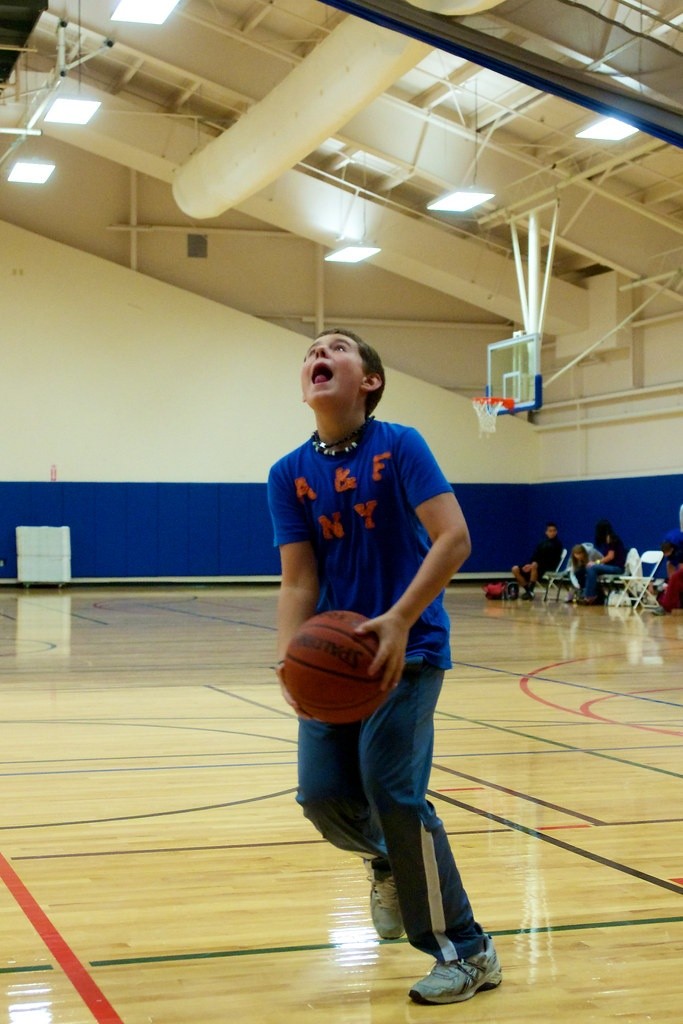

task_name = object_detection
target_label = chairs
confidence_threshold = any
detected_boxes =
[534,549,664,608]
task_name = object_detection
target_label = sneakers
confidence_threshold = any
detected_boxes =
[364,856,406,939]
[408,933,502,1003]
[652,607,665,615]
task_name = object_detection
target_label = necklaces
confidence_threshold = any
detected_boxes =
[310,414,374,457]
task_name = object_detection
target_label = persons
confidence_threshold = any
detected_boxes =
[566,520,627,603]
[267,330,504,1006]
[512,525,562,600]
[661,541,683,609]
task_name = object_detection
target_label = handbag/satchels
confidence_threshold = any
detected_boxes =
[482,582,507,600]
[608,589,632,606]
[504,581,519,601]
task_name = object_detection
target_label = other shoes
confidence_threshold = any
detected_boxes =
[576,597,585,604]
[521,591,535,600]
[564,588,575,602]
[584,595,605,605]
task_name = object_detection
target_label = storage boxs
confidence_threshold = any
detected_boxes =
[17,556,71,582]
[15,525,71,556]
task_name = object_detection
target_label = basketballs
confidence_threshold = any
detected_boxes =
[283,610,394,723]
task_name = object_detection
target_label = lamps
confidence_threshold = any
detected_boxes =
[110,0,177,29]
[573,115,636,141]
[43,0,101,125]
[313,196,383,263]
[425,79,496,215]
[5,44,55,185]
